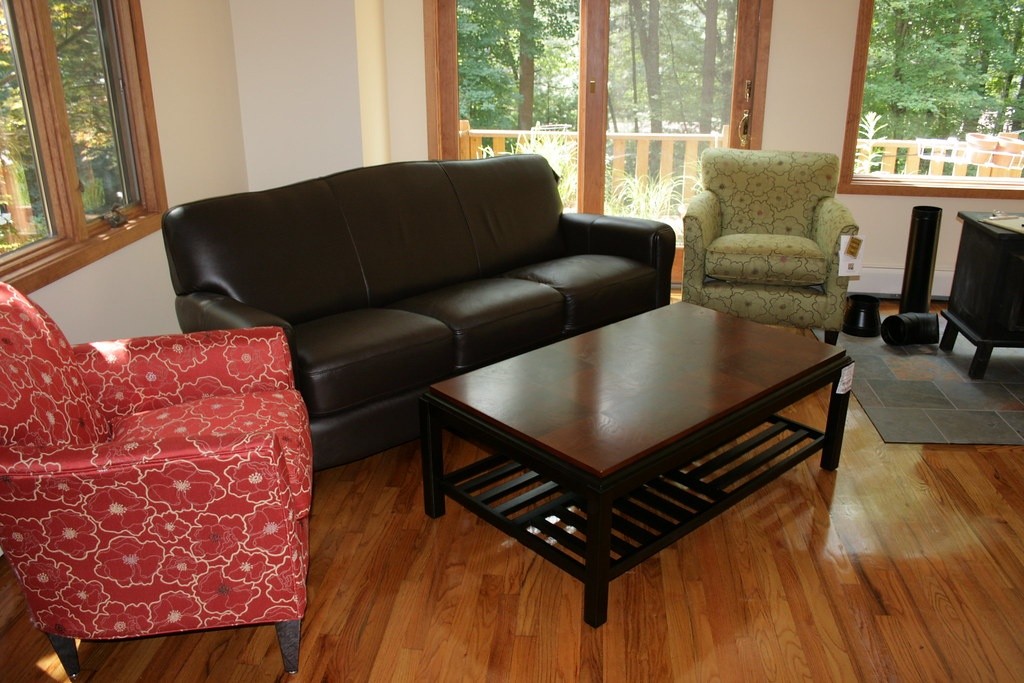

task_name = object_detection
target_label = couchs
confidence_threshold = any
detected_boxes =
[0,281,312,677]
[161,154,677,472]
[683,148,859,346]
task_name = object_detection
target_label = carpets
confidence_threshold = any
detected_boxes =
[811,316,1024,444]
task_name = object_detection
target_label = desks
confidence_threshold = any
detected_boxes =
[939,211,1024,379]
[418,301,855,629]
